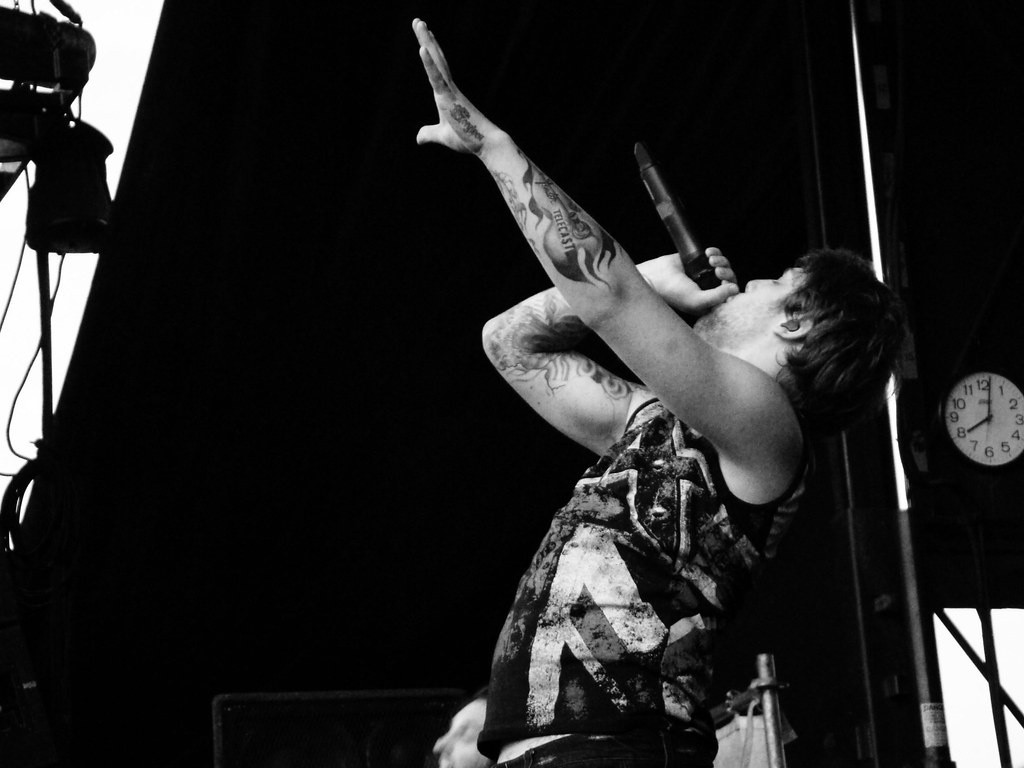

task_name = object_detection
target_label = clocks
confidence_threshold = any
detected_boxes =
[927,352,1023,478]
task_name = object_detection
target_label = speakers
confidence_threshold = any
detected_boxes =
[202,688,469,768]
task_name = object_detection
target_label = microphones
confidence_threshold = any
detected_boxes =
[635,141,721,291]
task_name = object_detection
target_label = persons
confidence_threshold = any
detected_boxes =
[408,12,908,768]
[430,685,499,768]
